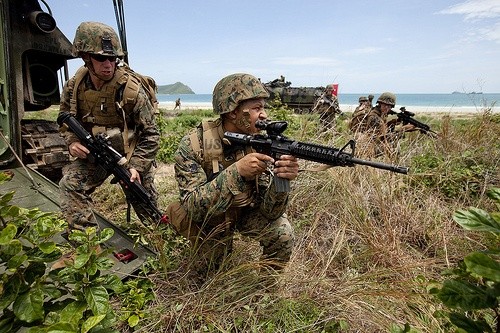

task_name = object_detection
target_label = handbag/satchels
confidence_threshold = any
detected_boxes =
[167,131,204,244]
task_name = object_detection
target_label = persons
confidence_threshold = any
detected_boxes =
[174,98,181,110]
[316,84,339,131]
[352,96,369,133]
[174,74,299,290]
[368,95,374,111]
[50,21,161,274]
[367,92,413,145]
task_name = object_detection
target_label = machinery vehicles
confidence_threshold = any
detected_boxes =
[264,75,339,114]
[0,0,158,289]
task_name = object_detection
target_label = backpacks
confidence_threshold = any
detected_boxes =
[68,61,159,131]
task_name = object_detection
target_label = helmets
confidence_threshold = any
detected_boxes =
[71,21,125,61]
[377,92,396,106]
[212,73,270,116]
[359,96,368,102]
[325,84,334,92]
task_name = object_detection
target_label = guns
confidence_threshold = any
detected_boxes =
[313,90,347,117]
[56,111,163,223]
[386,107,438,137]
[223,119,409,192]
